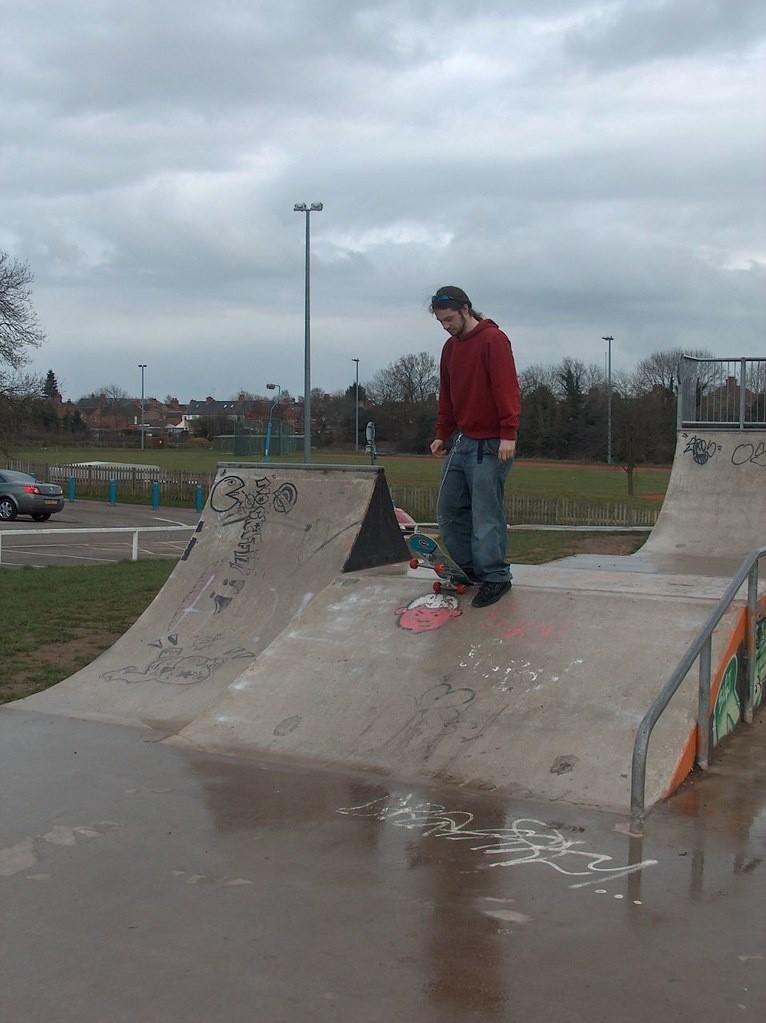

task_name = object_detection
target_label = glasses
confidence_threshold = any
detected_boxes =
[431,294,454,302]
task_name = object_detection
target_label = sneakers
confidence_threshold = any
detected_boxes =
[472,581,512,607]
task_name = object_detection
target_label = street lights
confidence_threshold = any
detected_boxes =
[293,200,325,464]
[601,335,614,465]
[137,364,146,449]
[351,357,360,451]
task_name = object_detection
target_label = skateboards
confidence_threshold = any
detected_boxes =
[406,533,476,594]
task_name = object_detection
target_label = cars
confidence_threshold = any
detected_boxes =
[0,467,65,522]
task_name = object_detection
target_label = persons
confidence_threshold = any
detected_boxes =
[430,286,522,607]
